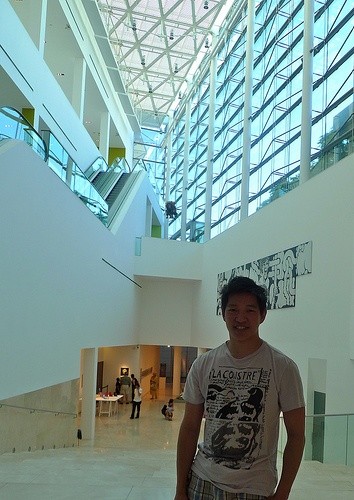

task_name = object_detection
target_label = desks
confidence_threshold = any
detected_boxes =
[96,395,124,418]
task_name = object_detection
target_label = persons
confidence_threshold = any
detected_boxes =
[174,276,306,500]
[115,372,175,421]
